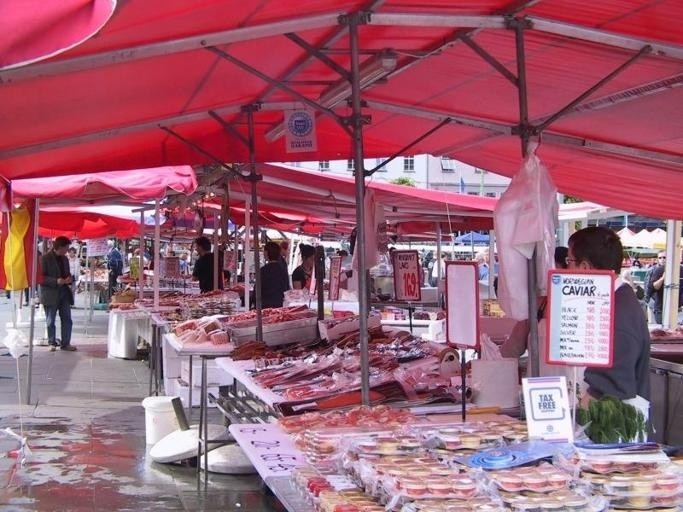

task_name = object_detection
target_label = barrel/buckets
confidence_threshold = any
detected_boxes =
[141,395,186,446]
[470,357,521,409]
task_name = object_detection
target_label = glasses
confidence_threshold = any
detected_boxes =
[564,257,576,265]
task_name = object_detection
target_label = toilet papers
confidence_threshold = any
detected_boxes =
[383,323,410,339]
[424,341,459,365]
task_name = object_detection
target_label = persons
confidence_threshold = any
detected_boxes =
[677,248,683,324]
[564,226,653,425]
[336,248,348,256]
[38,233,80,353]
[323,247,335,284]
[65,247,81,309]
[22,249,43,308]
[105,239,123,295]
[128,245,189,275]
[642,264,660,324]
[387,246,499,289]
[192,235,213,296]
[500,246,580,411]
[250,241,285,309]
[292,243,315,291]
[280,241,290,291]
[648,248,667,324]
[222,269,230,283]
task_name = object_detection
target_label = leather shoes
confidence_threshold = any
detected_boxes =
[61,345,76,351]
[49,344,56,351]
